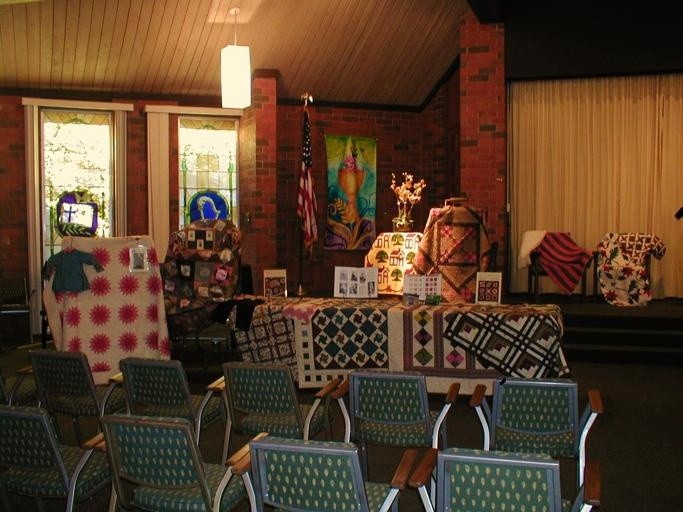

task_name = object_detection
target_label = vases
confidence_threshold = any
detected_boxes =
[393,217,414,233]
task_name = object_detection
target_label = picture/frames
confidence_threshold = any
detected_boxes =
[325,135,379,251]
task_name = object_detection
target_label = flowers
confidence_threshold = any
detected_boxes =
[391,174,427,217]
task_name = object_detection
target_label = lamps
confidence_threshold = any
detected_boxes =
[221,7,252,109]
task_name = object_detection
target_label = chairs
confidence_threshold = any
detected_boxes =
[40,237,167,380]
[523,231,587,300]
[120,356,226,452]
[410,449,602,512]
[594,232,665,307]
[212,362,339,466]
[333,371,461,466]
[31,348,125,441]
[472,378,604,496]
[0,405,105,512]
[102,415,249,512]
[231,436,416,512]
[155,220,242,350]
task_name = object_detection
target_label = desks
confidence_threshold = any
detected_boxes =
[227,298,566,404]
